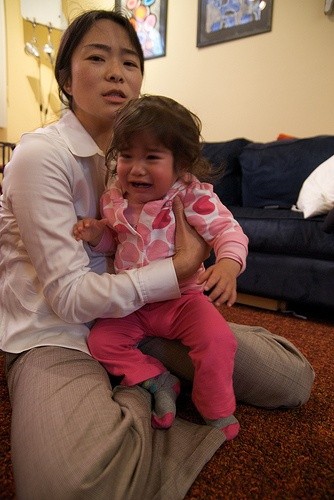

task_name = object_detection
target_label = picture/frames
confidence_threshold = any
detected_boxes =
[115,0,168,63]
[196,0,273,49]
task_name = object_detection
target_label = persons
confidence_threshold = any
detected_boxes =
[1,8,316,500]
[72,93,240,441]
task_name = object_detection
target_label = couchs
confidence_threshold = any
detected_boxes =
[177,298,334,500]
[193,134,333,326]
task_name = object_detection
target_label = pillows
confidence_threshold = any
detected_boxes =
[290,155,334,220]
